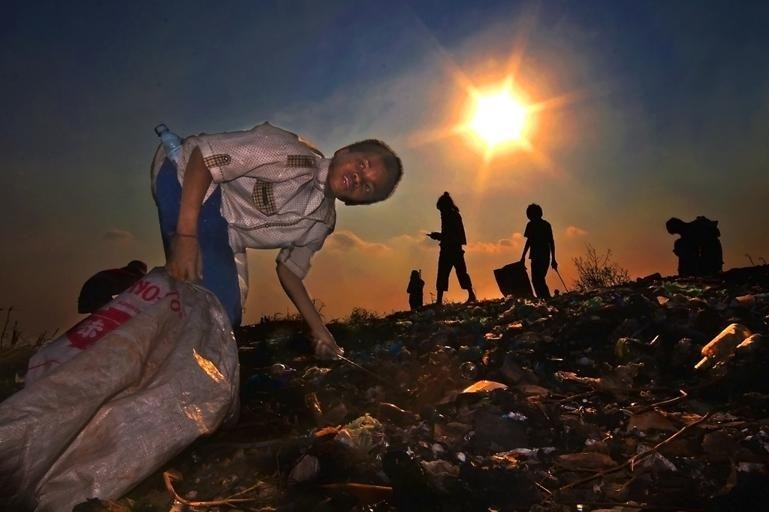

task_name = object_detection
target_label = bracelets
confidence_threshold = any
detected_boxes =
[174,229,201,244]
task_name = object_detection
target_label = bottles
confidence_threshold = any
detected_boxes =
[694,323,752,372]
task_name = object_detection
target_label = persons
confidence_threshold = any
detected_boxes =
[426,192,477,309]
[406,270,426,312]
[666,213,724,278]
[152,119,406,365]
[78,259,148,316]
[519,204,557,299]
